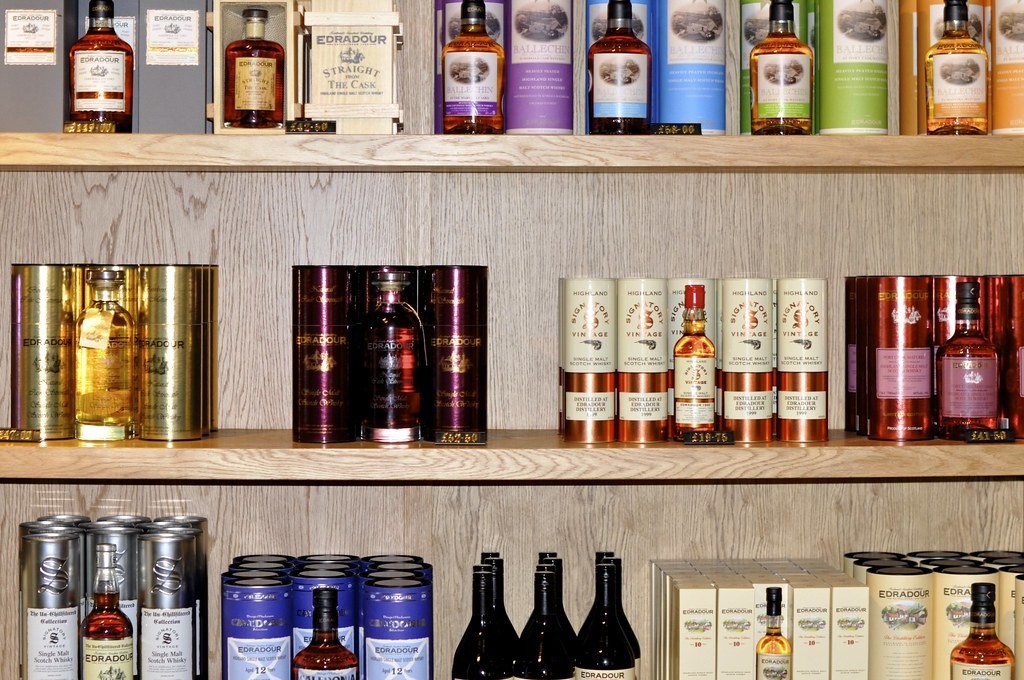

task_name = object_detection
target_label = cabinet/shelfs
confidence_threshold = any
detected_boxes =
[0,0,1024,678]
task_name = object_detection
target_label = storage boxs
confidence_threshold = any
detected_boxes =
[649,558,870,680]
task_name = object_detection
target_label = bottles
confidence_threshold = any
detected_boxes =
[587,1,652,134]
[935,281,999,441]
[63,0,133,135]
[750,1,813,135]
[79,544,132,680]
[574,551,642,680]
[291,587,358,680]
[442,0,506,135]
[364,270,428,442]
[925,0,990,135]
[673,285,717,442]
[223,9,284,129]
[756,586,792,680]
[949,581,1017,680]
[72,270,137,441]
[518,551,578,680]
[450,550,520,680]
